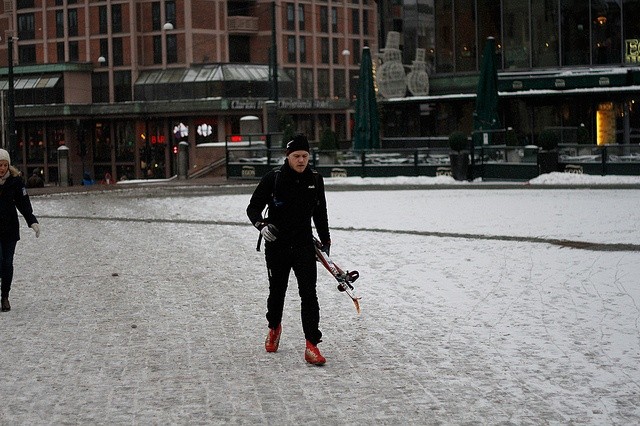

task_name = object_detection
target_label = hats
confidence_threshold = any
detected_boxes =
[0,149,10,166]
[286,135,310,156]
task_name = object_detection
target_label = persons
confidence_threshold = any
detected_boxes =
[246,136,331,367]
[0,149,40,312]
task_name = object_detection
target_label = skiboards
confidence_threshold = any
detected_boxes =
[311,236,363,315]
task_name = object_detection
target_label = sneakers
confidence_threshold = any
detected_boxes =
[1,296,11,312]
[265,323,282,352]
[305,339,327,366]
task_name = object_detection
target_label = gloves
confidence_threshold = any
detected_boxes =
[316,239,331,261]
[254,215,280,243]
[30,223,40,238]
